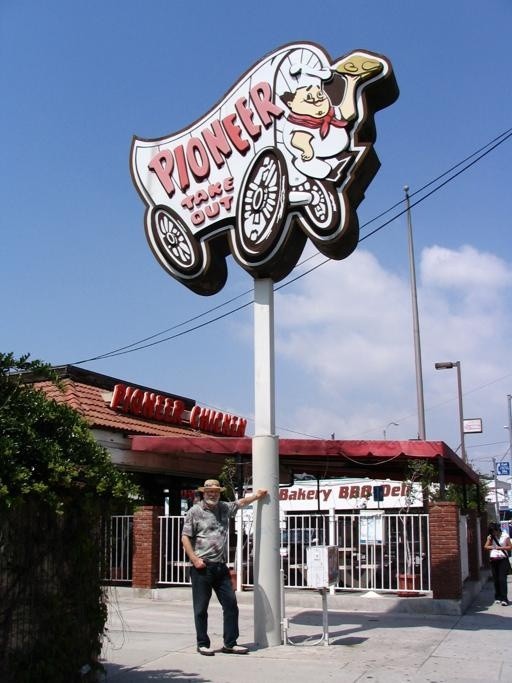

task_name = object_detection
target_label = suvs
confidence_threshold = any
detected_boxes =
[240,525,329,592]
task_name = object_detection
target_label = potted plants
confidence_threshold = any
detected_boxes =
[396,458,438,595]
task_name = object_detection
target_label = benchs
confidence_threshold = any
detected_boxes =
[173,541,380,588]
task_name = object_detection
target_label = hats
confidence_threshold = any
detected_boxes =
[198,479,227,492]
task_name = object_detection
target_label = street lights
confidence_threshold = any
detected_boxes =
[382,419,400,442]
[433,360,466,468]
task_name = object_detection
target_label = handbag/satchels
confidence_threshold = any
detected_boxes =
[506,559,512,574]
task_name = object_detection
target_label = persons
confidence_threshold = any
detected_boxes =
[182,476,269,656]
[484,521,512,605]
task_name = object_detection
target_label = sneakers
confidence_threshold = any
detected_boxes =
[197,645,214,656]
[493,599,500,604]
[223,644,249,653]
[501,600,508,605]
